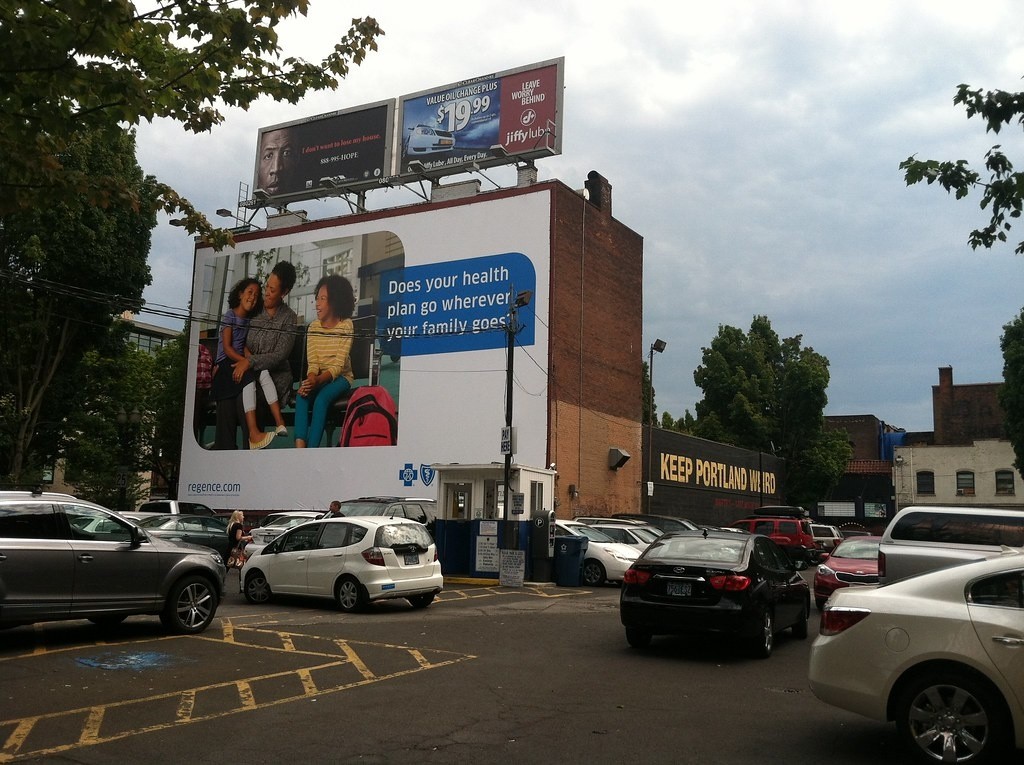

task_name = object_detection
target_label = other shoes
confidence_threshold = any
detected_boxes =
[249,431,276,449]
[276,425,288,436]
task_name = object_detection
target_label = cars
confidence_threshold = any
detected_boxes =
[402,123,456,159]
[211,510,444,613]
[619,527,810,659]
[555,512,754,587]
[814,535,882,610]
[806,505,1024,765]
[0,483,232,634]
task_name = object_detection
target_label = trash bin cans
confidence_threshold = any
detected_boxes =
[551,534,589,587]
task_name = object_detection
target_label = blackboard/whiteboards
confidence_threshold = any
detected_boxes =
[499,550,526,588]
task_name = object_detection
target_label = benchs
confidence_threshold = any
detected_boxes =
[198,314,377,449]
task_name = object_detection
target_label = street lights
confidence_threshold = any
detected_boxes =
[648,339,667,515]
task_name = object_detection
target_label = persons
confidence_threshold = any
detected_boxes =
[226,510,252,554]
[259,127,298,195]
[294,275,354,448]
[329,500,345,518]
[215,261,296,450]
[215,277,288,449]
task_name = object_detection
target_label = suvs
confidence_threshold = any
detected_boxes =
[320,496,437,541]
[727,505,872,566]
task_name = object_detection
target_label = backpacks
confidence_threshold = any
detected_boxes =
[339,385,398,447]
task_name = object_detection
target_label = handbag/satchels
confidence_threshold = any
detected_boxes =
[226,536,248,571]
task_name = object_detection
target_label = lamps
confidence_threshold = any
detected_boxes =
[389,176,429,200]
[489,144,518,172]
[216,209,262,229]
[325,188,366,212]
[169,219,208,236]
[252,188,308,221]
[408,160,441,186]
[461,162,501,190]
[318,176,367,213]
